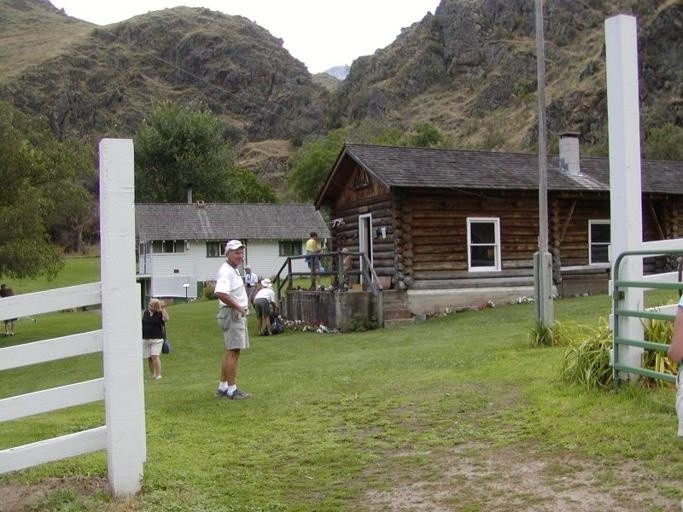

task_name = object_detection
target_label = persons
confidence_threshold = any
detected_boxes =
[214,239,276,399]
[0,284,17,336]
[342,247,352,291]
[142,298,169,380]
[667,293,683,437]
[305,233,323,290]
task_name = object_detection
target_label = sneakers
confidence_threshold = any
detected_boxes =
[225,389,249,400]
[213,388,228,398]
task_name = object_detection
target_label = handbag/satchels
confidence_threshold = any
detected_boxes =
[161,342,169,354]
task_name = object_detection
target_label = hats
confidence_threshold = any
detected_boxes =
[260,278,273,288]
[223,239,246,256]
